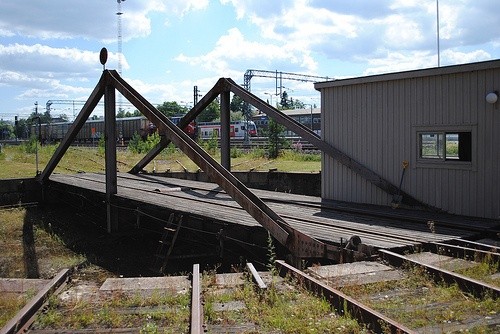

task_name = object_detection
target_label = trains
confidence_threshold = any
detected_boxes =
[30,115,257,140]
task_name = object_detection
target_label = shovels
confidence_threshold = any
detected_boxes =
[392,161,409,210]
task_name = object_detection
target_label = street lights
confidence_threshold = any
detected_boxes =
[264,92,278,106]
[302,103,313,130]
